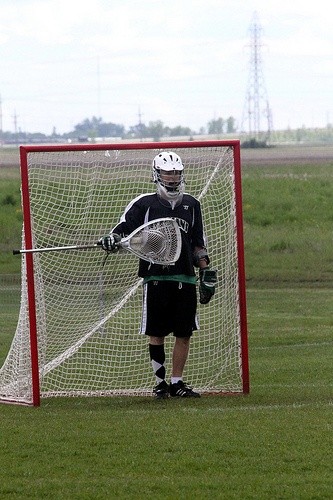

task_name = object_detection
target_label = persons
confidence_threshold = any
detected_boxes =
[95,151,218,399]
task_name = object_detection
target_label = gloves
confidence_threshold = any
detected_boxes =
[97,233,121,253]
[197,267,218,304]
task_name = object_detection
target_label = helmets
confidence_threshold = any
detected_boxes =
[152,151,186,198]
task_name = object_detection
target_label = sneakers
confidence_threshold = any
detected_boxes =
[152,381,169,394]
[169,380,200,398]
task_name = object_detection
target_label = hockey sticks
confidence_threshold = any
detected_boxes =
[12,217,182,266]
